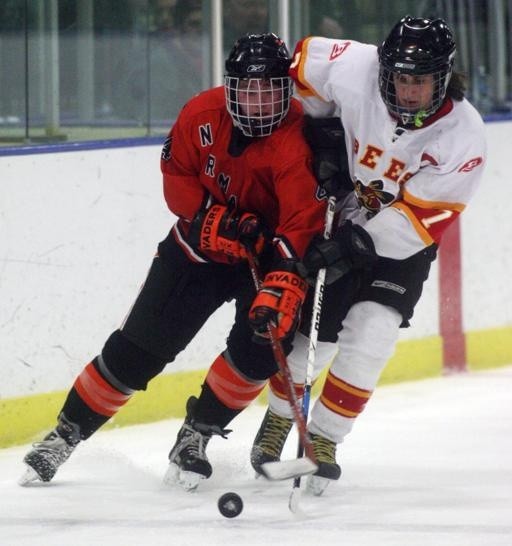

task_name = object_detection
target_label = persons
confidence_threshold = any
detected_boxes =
[22,31,327,483]
[249,16,486,479]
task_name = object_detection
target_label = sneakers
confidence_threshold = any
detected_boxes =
[307,432,340,480]
[251,409,294,476]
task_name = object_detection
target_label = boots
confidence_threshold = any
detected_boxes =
[25,412,81,482]
[169,396,232,479]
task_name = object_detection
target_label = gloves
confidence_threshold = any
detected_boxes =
[297,220,378,302]
[248,257,306,349]
[302,113,354,202]
[188,205,266,266]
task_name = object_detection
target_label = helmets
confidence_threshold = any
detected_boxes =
[377,14,455,127]
[222,32,294,141]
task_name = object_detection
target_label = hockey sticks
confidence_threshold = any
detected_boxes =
[288,266,344,518]
[246,233,321,483]
